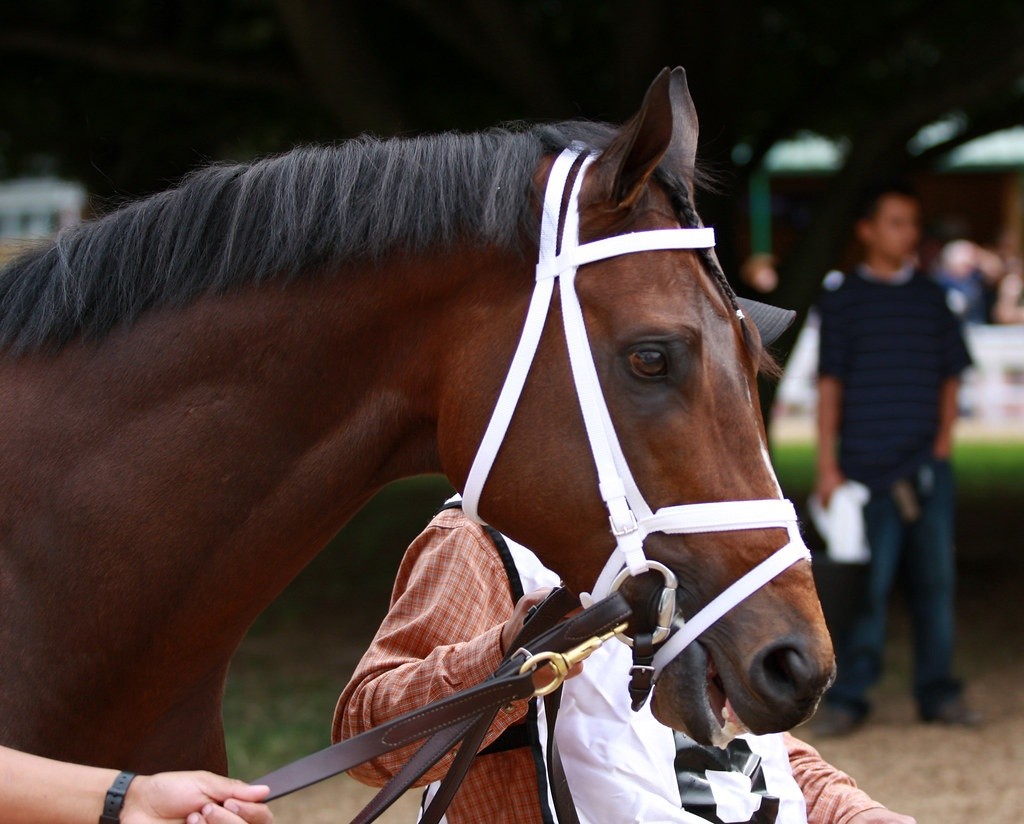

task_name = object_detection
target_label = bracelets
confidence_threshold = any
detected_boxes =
[98,770,140,824]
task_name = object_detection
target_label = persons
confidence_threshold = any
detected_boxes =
[1,745,275,824]
[332,297,918,824]
[738,186,1023,738]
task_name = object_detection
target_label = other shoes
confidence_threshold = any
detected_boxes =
[816,699,864,736]
[917,696,978,725]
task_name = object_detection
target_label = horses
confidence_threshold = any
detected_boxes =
[0,67,835,824]
